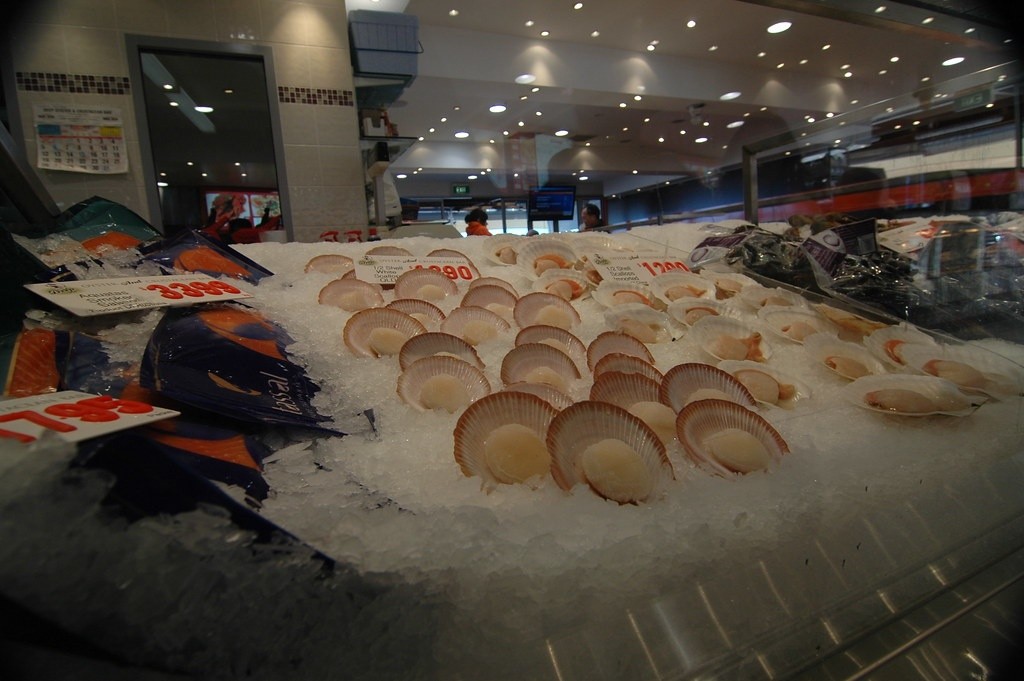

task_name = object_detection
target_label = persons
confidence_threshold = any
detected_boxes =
[579,203,610,234]
[465,209,491,237]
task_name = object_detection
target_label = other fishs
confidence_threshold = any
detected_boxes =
[715,211,1024,353]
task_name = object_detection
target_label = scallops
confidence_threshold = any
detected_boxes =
[308,234,1024,506]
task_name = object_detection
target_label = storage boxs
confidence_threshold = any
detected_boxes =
[361,108,386,137]
[349,10,424,111]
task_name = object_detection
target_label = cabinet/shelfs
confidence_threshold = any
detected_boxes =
[355,70,419,173]
